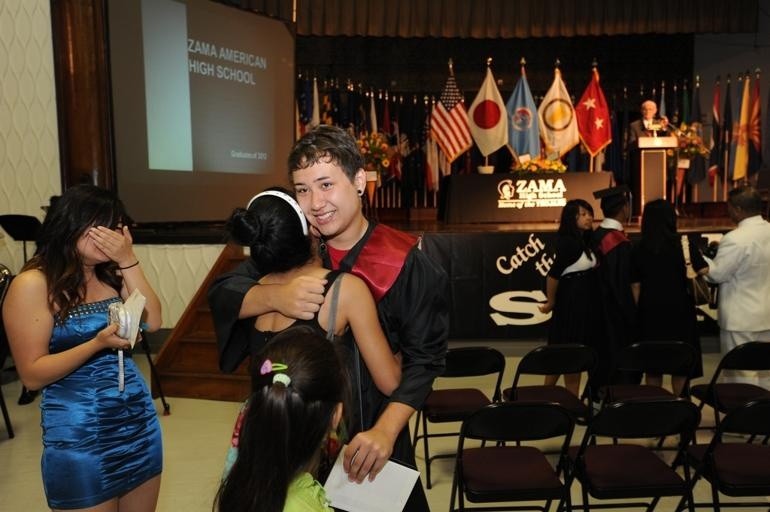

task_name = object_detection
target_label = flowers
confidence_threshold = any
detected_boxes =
[666,122,709,158]
[508,152,569,176]
[356,129,391,172]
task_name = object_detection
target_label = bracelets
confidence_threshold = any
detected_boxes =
[119,261,139,269]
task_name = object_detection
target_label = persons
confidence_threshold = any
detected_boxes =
[1,184,162,512]
[211,324,348,512]
[538,199,603,399]
[207,124,450,512]
[635,199,709,396]
[581,185,644,415]
[222,185,403,477]
[703,186,770,440]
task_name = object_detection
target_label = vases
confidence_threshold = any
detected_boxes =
[673,158,689,197]
[363,169,376,205]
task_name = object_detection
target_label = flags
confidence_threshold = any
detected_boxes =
[467,65,509,157]
[505,66,541,163]
[424,70,473,164]
[609,72,763,195]
[537,68,580,160]
[295,76,451,209]
[575,67,613,156]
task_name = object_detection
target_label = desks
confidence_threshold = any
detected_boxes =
[437,172,615,226]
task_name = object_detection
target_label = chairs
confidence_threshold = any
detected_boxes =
[0,259,18,444]
[411,341,770,512]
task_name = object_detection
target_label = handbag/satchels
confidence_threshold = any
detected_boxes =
[679,234,714,307]
